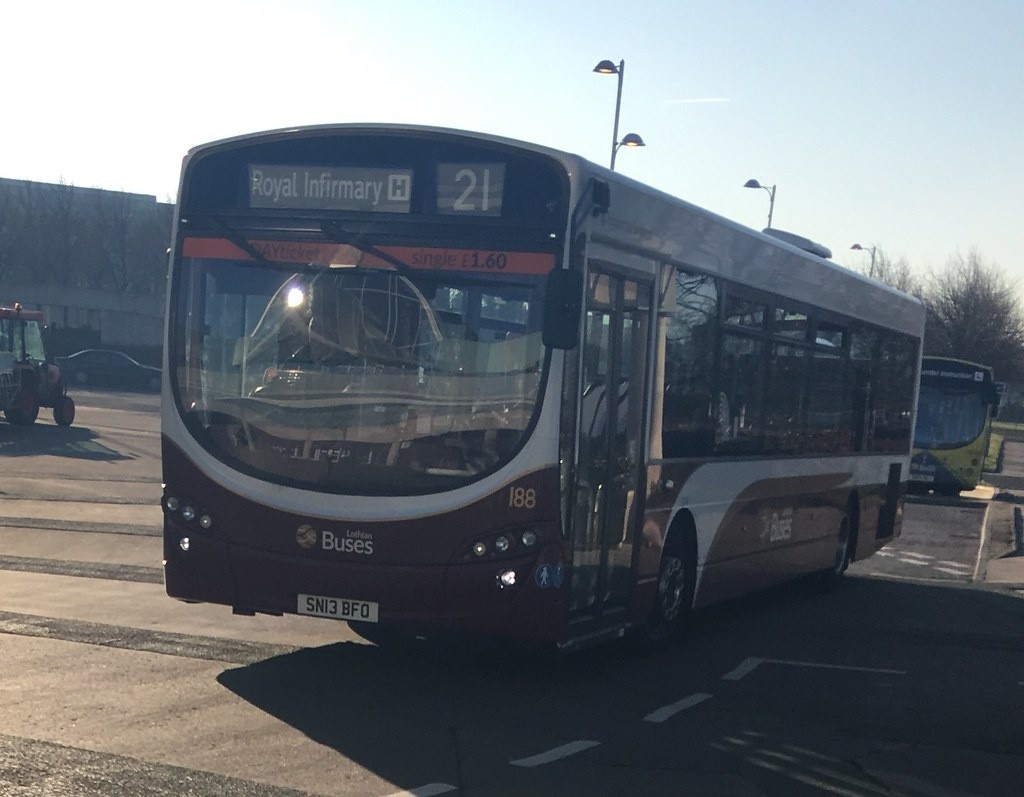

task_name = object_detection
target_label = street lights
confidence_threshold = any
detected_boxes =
[745,171,778,239]
[593,57,646,174]
[849,241,878,282]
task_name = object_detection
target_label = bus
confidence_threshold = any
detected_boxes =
[902,359,1001,497]
[153,115,927,659]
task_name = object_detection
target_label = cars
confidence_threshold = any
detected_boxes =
[53,349,162,394]
[0,302,78,431]
